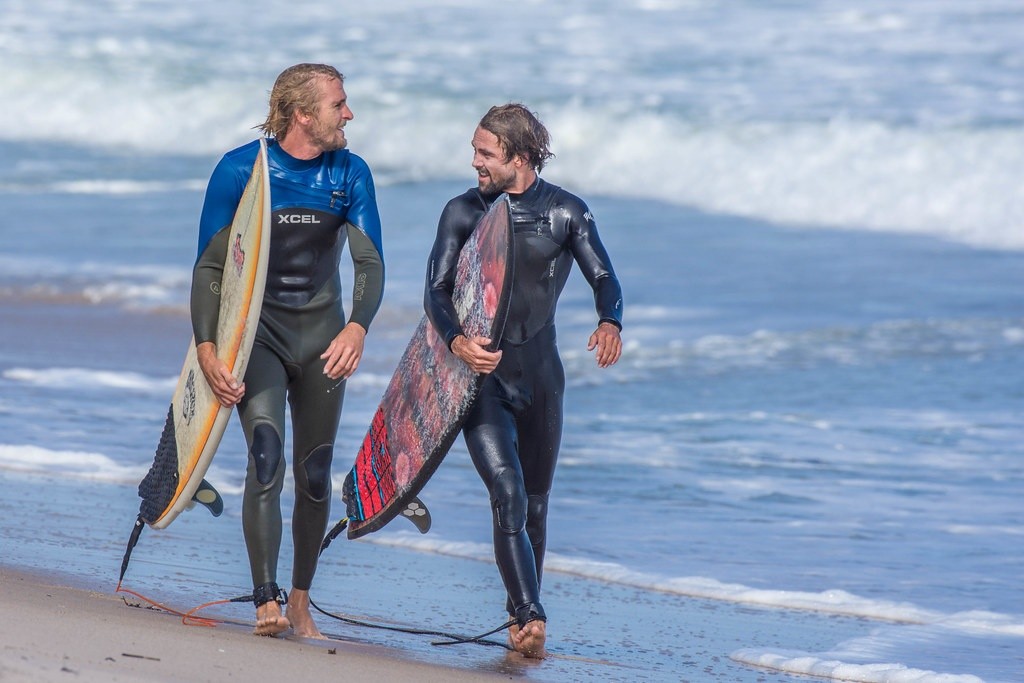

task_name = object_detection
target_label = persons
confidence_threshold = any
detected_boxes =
[423,103,626,660]
[189,62,387,641]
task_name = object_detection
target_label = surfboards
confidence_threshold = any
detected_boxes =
[341,192,517,540]
[138,138,271,532]
